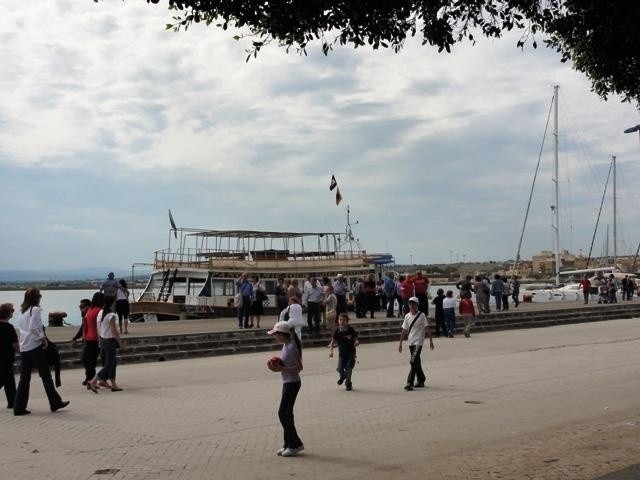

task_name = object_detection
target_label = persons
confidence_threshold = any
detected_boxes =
[304,274,347,347]
[353,272,430,319]
[236,272,266,329]
[578,274,591,304]
[266,321,304,457]
[599,274,617,303]
[399,297,434,390]
[275,277,304,338]
[457,274,521,312]
[0,271,130,416]
[431,288,457,337]
[622,275,640,301]
[329,314,359,391]
[459,292,475,337]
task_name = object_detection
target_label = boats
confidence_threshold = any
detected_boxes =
[122,202,397,324]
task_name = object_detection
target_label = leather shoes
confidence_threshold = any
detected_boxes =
[51,401,70,412]
[14,410,31,415]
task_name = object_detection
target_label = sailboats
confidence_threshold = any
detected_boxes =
[494,79,640,308]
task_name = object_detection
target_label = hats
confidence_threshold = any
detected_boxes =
[266,320,292,335]
[409,297,419,304]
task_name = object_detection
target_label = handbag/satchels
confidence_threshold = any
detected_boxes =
[326,308,337,320]
[256,283,268,300]
[43,340,59,366]
[234,285,244,309]
[284,305,290,321]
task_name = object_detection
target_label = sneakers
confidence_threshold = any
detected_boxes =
[276,447,285,455]
[281,445,304,456]
[337,377,345,385]
[413,382,424,387]
[345,384,352,390]
[404,383,413,391]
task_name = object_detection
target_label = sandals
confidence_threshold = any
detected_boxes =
[82,380,123,394]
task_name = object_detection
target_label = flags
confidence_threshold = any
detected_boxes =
[329,174,342,205]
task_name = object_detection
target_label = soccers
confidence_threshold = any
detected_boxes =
[268,356,283,371]
